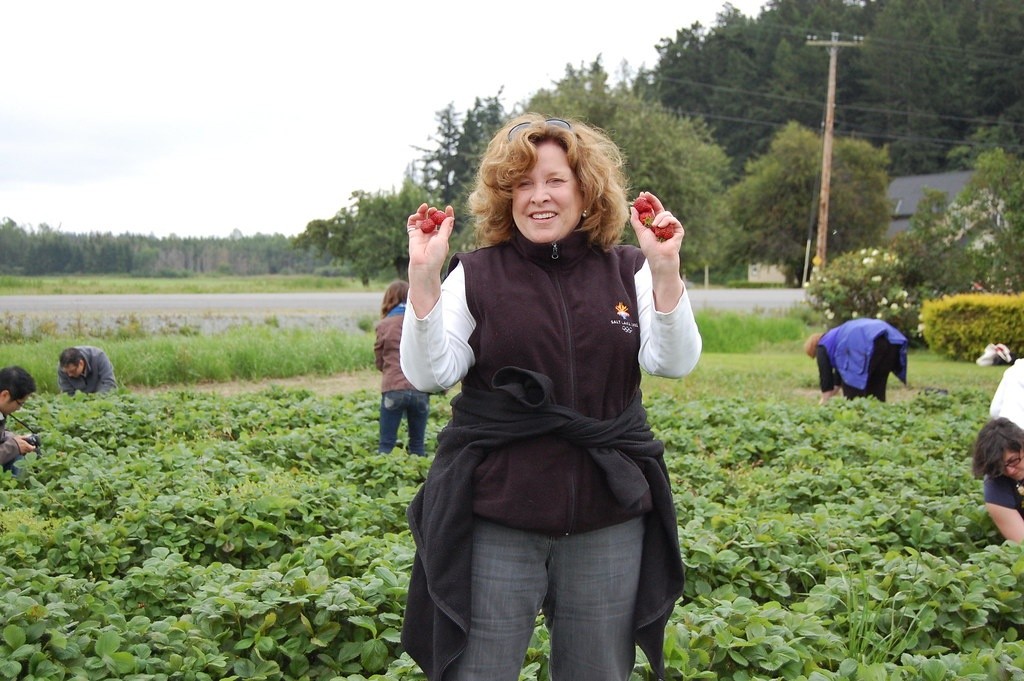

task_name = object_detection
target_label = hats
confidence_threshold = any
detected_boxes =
[805,334,821,359]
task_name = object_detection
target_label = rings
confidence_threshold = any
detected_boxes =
[407,228,416,233]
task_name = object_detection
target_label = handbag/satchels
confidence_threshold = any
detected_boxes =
[976,343,1012,366]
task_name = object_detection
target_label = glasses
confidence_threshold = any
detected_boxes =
[15,399,25,408]
[66,366,78,374]
[508,118,573,143]
[1004,451,1021,471]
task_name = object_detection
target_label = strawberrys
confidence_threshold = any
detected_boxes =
[430,210,447,225]
[421,218,435,233]
[633,197,674,241]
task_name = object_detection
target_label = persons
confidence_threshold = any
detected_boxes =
[0,366,35,478]
[973,417,1024,543]
[400,115,702,681]
[373,281,431,456]
[58,346,118,397]
[989,358,1024,429]
[805,319,908,405]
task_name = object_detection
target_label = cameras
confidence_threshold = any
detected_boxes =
[23,435,41,447]
